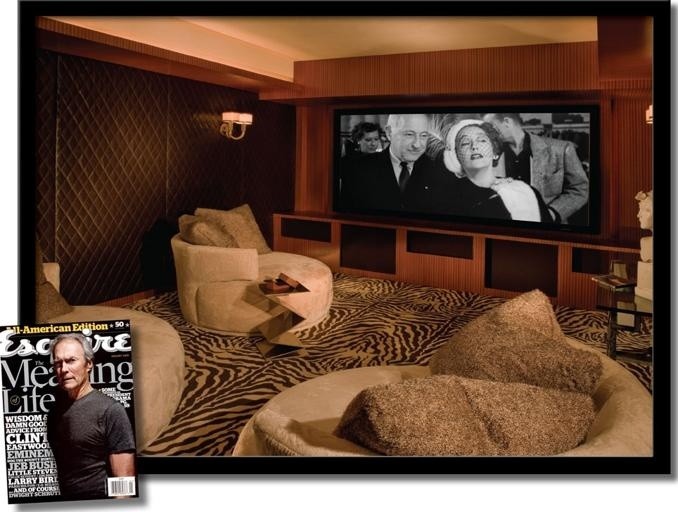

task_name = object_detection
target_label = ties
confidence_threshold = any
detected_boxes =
[398,162,410,200]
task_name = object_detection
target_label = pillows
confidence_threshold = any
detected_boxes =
[35,233,73,326]
[193,204,271,255]
[332,375,594,458]
[429,289,602,396]
[178,214,240,249]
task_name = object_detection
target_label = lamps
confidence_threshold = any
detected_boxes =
[220,112,254,140]
[645,104,653,125]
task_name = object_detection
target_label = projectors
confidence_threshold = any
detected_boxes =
[221,110,254,127]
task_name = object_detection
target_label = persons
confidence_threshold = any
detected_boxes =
[364,113,439,215]
[634,189,653,237]
[339,121,381,199]
[46,334,135,499]
[442,118,554,224]
[483,113,589,226]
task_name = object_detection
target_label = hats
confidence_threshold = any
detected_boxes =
[443,120,486,175]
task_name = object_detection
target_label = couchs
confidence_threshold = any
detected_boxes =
[171,203,333,336]
[231,335,654,461]
[35,263,184,456]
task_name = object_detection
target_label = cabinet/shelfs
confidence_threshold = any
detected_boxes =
[272,209,641,312]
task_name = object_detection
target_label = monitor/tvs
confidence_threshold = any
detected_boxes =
[331,103,601,235]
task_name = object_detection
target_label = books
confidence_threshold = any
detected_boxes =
[591,273,637,287]
